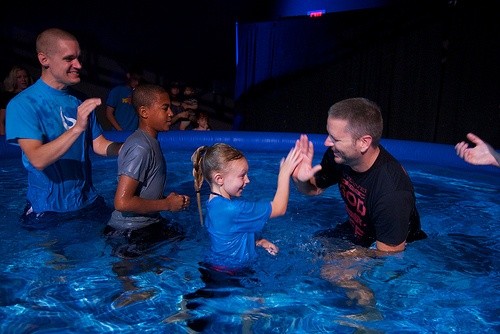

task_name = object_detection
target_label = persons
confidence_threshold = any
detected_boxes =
[4,29,125,272]
[104,82,189,309]
[181,143,304,334]
[454,132,500,165]
[291,98,428,322]
[0,68,211,131]
